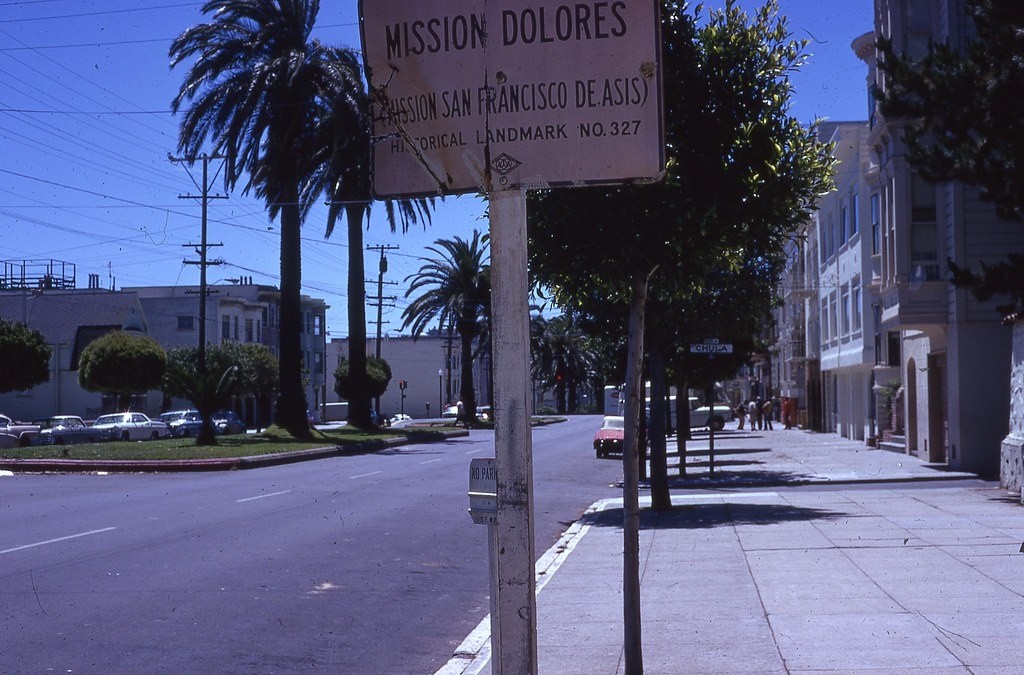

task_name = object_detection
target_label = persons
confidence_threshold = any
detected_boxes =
[452,397,470,429]
[735,396,793,430]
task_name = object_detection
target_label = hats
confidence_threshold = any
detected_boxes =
[757,396,761,400]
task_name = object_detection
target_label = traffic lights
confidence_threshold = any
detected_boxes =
[404,380,407,388]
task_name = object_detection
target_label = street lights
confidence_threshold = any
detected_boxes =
[376,328,400,415]
[199,278,240,445]
[439,369,442,418]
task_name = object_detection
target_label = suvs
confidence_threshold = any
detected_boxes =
[645,397,732,431]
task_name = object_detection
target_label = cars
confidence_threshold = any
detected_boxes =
[212,411,247,435]
[88,412,168,441]
[443,405,458,418]
[0,414,41,448]
[594,416,624,458]
[476,406,490,417]
[40,416,102,445]
[157,410,203,438]
